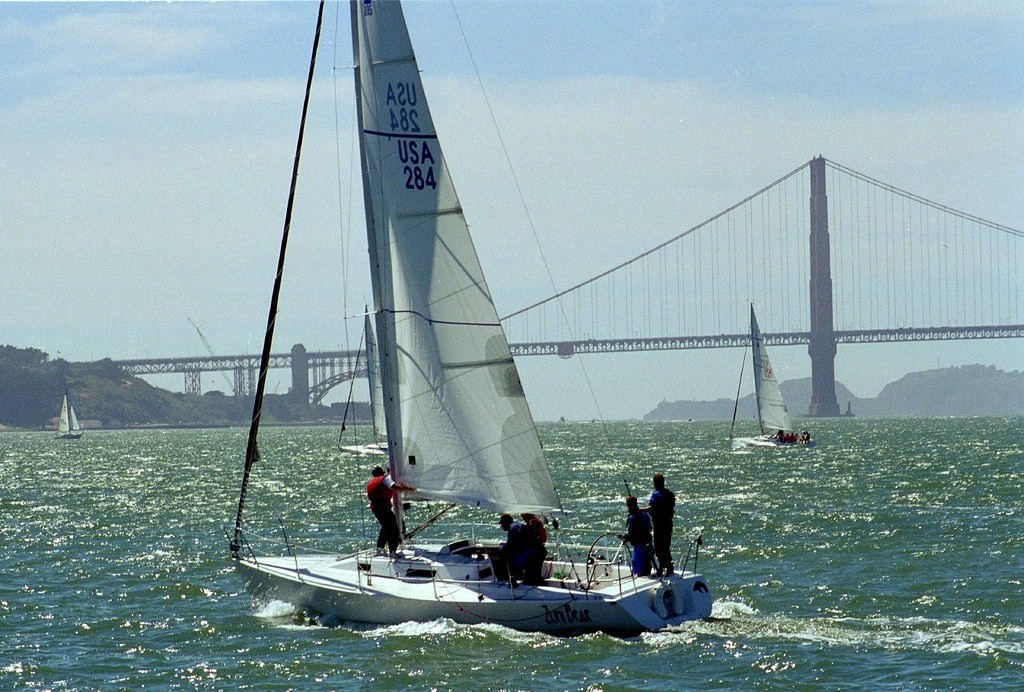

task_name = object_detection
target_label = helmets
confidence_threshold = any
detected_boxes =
[372,466,385,477]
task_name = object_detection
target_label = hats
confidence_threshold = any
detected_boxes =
[499,514,514,524]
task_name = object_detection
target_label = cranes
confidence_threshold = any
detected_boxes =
[188,317,248,399]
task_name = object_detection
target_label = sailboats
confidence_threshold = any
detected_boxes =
[54,387,84,439]
[336,302,388,455]
[724,301,818,448]
[227,1,714,638]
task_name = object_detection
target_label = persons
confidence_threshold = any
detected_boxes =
[638,473,675,577]
[617,496,654,576]
[491,512,547,584]
[775,428,811,446]
[366,466,416,558]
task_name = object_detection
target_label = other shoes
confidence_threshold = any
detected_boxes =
[649,572,662,579]
[665,566,674,578]
[509,581,518,589]
[390,550,402,559]
[376,550,386,556]
